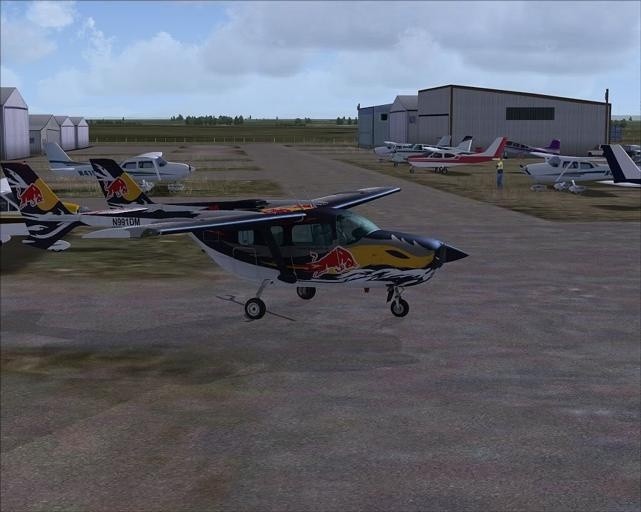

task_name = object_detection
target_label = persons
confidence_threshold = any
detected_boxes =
[496,158,504,186]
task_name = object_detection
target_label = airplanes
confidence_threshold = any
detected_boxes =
[372,136,641,199]
